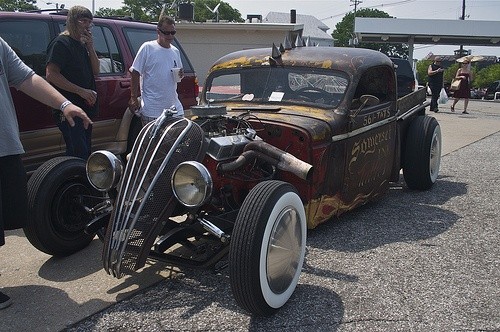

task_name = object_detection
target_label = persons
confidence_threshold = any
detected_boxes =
[428,56,444,113]
[128,15,185,154]
[46,6,100,160]
[0,37,93,311]
[451,61,474,114]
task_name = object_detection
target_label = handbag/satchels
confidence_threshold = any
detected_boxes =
[451,79,462,90]
[437,87,448,104]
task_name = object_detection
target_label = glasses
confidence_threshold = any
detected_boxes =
[77,19,94,28]
[160,29,177,36]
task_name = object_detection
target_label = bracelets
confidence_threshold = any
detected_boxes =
[59,100,71,112]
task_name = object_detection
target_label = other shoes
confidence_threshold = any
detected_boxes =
[451,105,454,112]
[462,111,469,114]
[430,104,439,113]
[0,291,12,310]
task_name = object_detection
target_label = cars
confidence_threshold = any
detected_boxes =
[418,81,455,98]
[470,80,500,100]
[22,33,442,318]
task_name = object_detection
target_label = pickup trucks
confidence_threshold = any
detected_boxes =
[0,12,201,195]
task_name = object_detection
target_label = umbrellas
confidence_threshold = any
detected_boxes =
[456,55,484,64]
